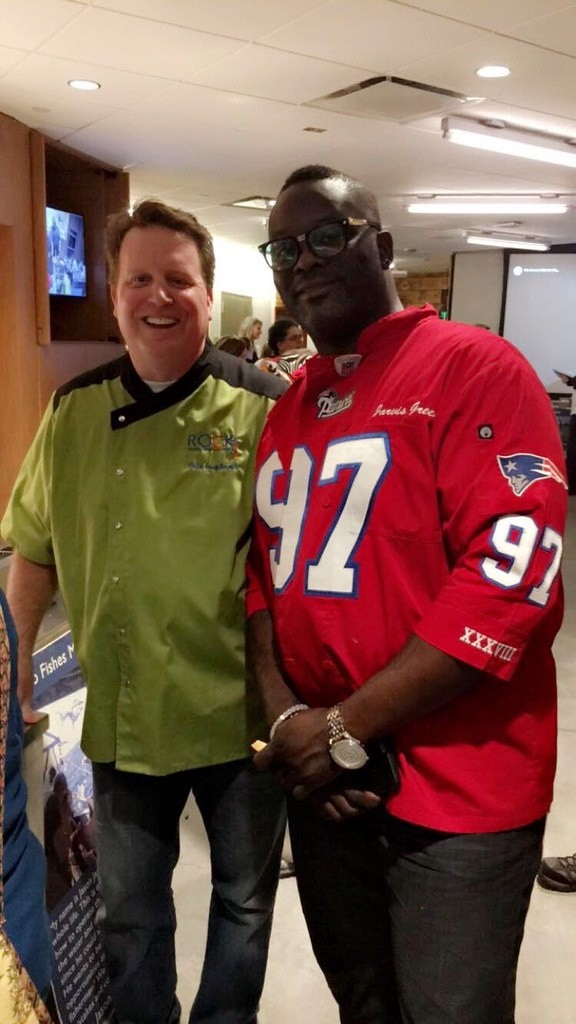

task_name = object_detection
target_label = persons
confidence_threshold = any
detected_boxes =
[269,319,304,354]
[1,200,287,1023]
[242,165,569,1024]
[561,372,576,496]
[43,742,96,907]
[213,336,247,361]
[238,316,263,363]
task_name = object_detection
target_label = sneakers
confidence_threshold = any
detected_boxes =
[537,853,576,893]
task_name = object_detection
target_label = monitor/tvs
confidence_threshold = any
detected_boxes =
[46,205,89,301]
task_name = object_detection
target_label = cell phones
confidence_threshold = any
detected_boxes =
[320,742,400,798]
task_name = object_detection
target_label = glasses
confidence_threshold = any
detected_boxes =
[285,334,303,341]
[257,216,381,274]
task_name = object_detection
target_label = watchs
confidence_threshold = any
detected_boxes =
[325,700,368,769]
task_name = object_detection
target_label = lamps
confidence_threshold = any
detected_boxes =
[466,230,554,254]
[441,116,576,171]
[402,195,569,217]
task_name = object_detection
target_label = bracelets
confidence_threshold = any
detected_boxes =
[269,704,309,740]
